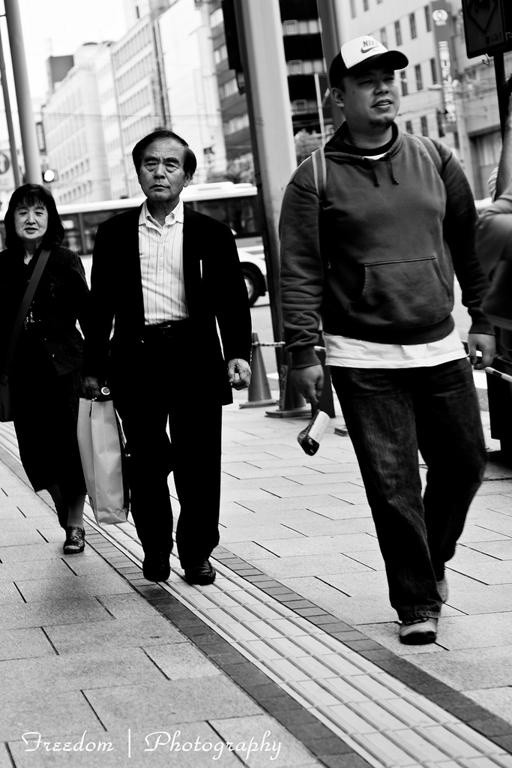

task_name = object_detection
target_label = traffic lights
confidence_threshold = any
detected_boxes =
[42,170,55,183]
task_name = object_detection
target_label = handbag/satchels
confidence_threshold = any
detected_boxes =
[76,395,132,526]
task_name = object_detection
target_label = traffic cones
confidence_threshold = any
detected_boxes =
[239,331,277,408]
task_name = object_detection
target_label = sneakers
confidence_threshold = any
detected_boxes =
[398,600,436,645]
[180,554,214,583]
[433,577,449,604]
[141,548,171,583]
[62,526,84,554]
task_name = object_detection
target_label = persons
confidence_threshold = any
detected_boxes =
[0,184,100,563]
[278,36,501,648]
[81,123,256,586]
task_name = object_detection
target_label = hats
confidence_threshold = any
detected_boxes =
[326,32,410,83]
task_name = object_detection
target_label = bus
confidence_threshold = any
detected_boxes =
[0,181,269,310]
